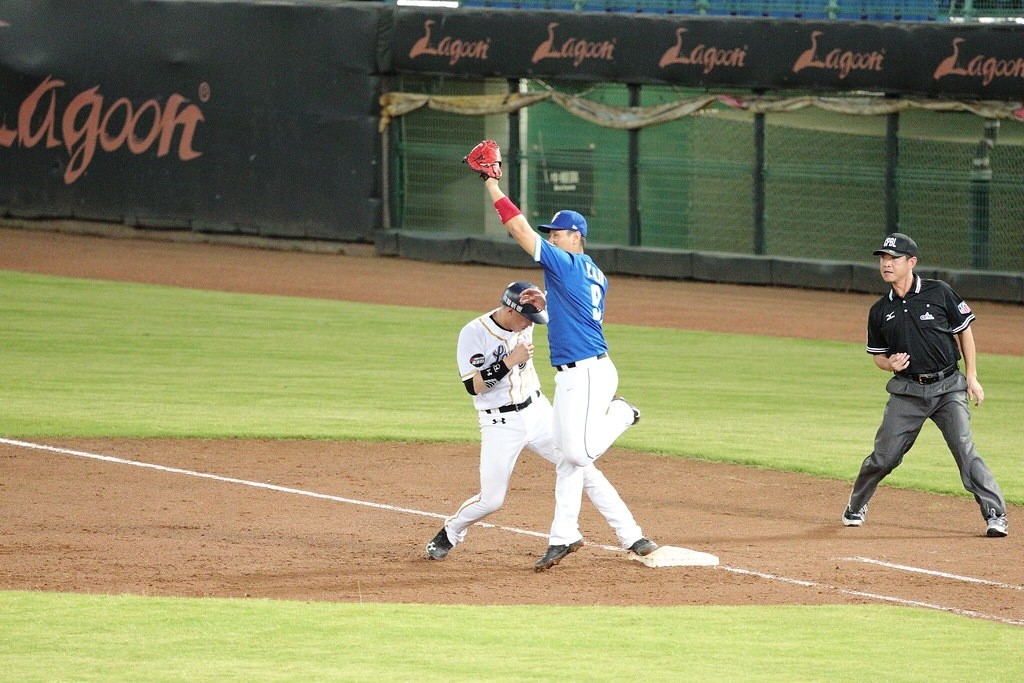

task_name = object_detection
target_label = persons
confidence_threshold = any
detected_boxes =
[425,282,658,561]
[841,233,1009,537]
[460,139,641,574]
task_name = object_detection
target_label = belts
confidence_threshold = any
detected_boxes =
[896,365,955,385]
[485,390,540,413]
[556,352,606,371]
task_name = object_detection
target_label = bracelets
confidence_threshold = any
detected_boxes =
[480,360,510,388]
[493,197,522,225]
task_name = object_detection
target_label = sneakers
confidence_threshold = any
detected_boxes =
[425,526,453,559]
[535,539,583,569]
[624,538,658,556]
[986,508,1008,536]
[612,396,640,425]
[841,501,868,526]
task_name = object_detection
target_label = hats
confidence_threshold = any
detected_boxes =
[501,282,549,324]
[873,233,918,257]
[537,210,587,238]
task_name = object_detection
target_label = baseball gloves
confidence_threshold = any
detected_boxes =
[461,139,503,183]
